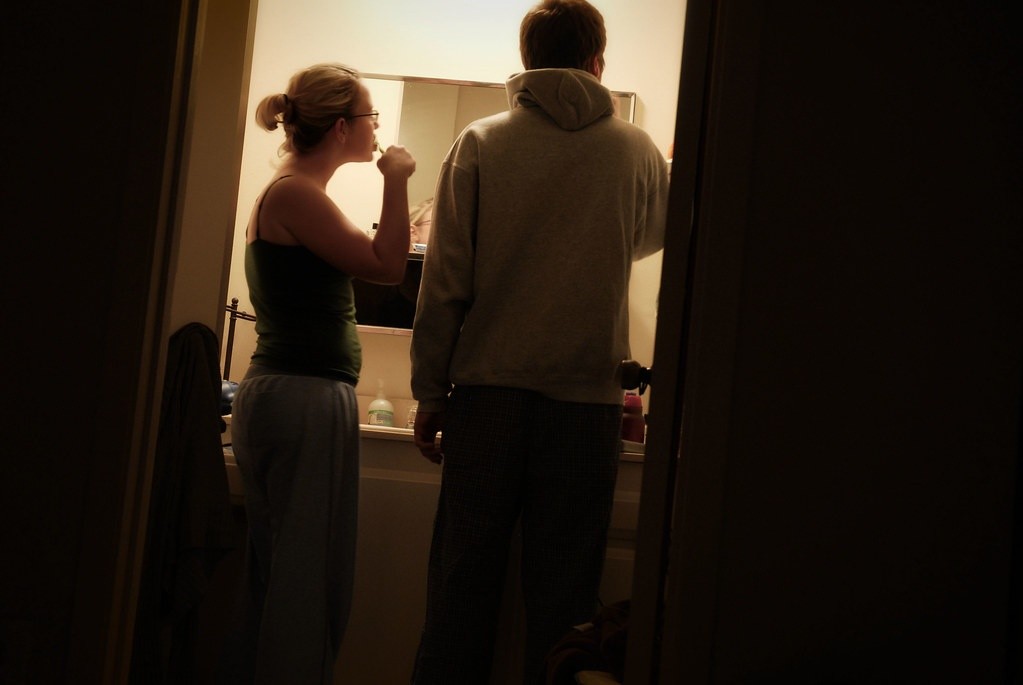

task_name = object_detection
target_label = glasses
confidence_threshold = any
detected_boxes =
[351,110,379,123]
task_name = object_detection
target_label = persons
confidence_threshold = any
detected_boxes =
[230,61,417,685]
[409,0,670,685]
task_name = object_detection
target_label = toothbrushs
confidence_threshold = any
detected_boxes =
[373,138,386,154]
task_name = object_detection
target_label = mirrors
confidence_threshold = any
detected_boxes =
[325,71,636,263]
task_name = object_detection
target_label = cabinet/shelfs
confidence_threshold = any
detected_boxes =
[330,428,521,685]
[593,451,645,619]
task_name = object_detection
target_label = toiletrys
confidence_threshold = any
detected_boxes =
[366,377,395,428]
